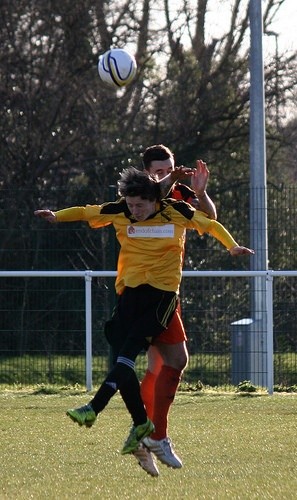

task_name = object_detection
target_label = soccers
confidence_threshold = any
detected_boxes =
[98,49,137,87]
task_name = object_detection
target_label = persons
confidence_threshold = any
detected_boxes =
[130,145,217,476]
[34,168,256,455]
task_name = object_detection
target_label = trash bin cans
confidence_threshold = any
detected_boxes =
[229,317,253,384]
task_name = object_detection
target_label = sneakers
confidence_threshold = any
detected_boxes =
[121,419,185,477]
[68,405,97,428]
